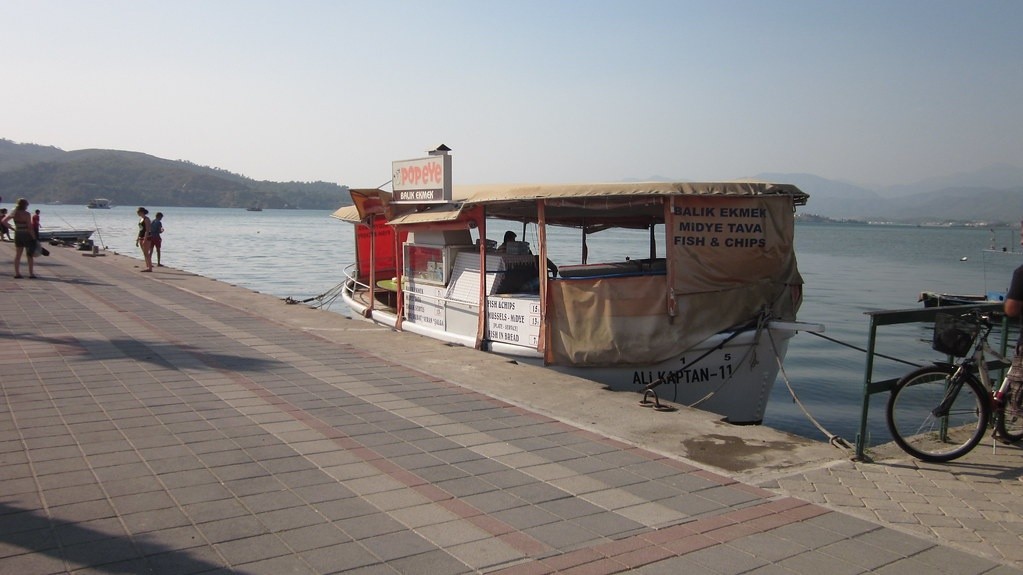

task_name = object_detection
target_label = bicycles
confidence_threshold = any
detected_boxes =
[884,308,1022,464]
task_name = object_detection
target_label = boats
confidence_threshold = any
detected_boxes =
[85,197,114,210]
[328,144,828,426]
[916,287,1022,325]
[3,221,97,241]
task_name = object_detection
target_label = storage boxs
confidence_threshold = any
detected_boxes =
[402,241,475,287]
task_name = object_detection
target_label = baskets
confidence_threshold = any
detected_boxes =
[931,312,982,358]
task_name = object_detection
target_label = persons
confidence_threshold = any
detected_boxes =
[498,231,517,251]
[548,258,557,277]
[0,197,13,241]
[1,198,37,279]
[1005,221,1023,484]
[33,210,40,239]
[149,213,164,267]
[136,207,152,272]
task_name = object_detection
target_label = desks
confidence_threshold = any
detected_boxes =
[377,279,404,307]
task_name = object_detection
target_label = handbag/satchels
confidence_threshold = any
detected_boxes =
[30,239,41,257]
